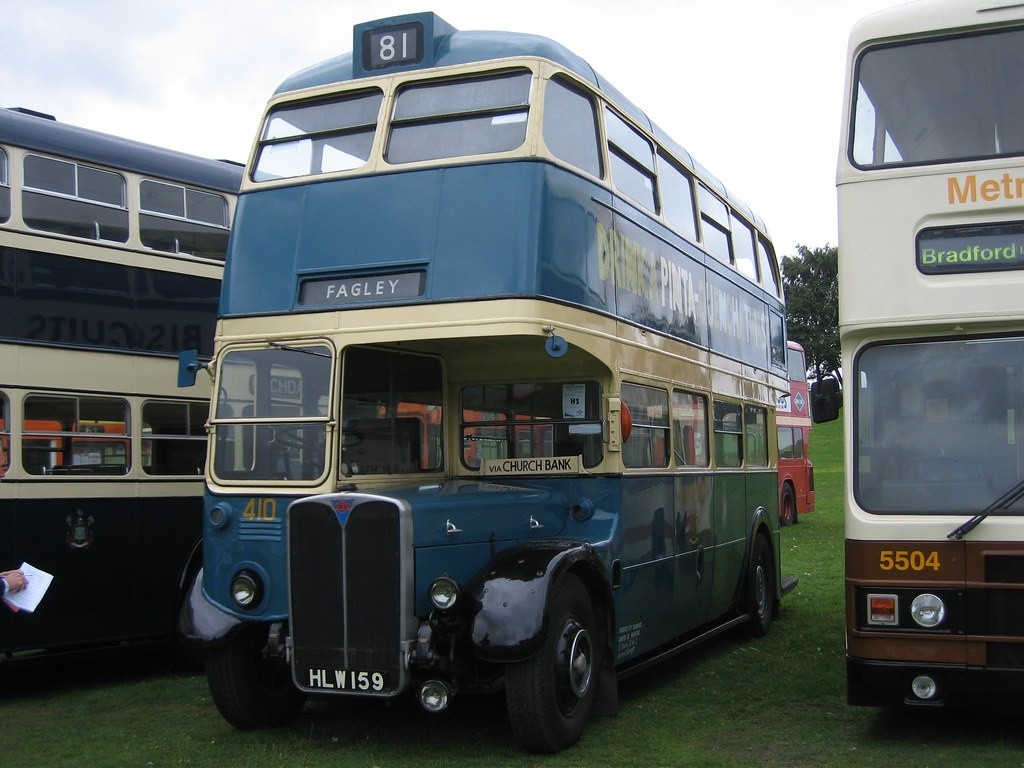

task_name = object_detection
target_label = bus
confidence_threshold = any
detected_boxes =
[177,12,814,754]
[0,106,249,667]
[810,0,1024,710]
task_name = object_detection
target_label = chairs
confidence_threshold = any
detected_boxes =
[880,455,993,506]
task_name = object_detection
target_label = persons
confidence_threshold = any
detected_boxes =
[0,569,29,598]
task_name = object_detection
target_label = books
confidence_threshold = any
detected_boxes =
[4,562,54,612]
